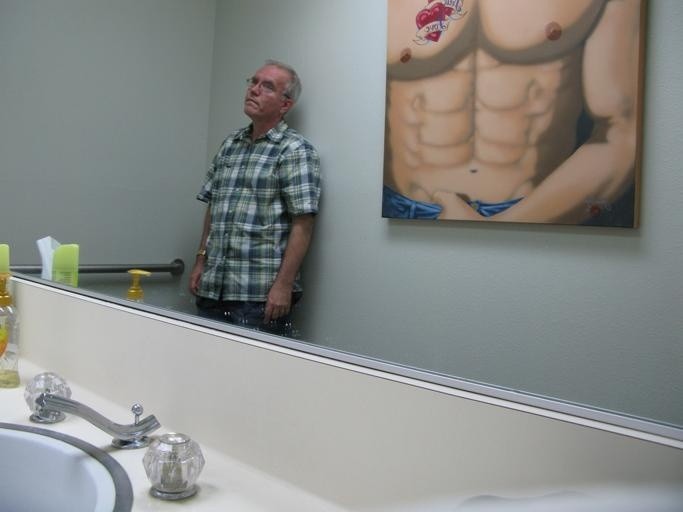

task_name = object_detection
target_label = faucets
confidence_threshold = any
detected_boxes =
[35,390,161,450]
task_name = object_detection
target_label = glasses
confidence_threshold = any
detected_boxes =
[246,78,291,100]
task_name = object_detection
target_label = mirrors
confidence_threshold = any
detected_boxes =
[1,1,683,454]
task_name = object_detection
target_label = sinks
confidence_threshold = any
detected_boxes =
[0,422,134,511]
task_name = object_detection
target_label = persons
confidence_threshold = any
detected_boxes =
[383,0,638,224]
[188,61,320,341]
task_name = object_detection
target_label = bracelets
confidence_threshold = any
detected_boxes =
[196,249,208,257]
